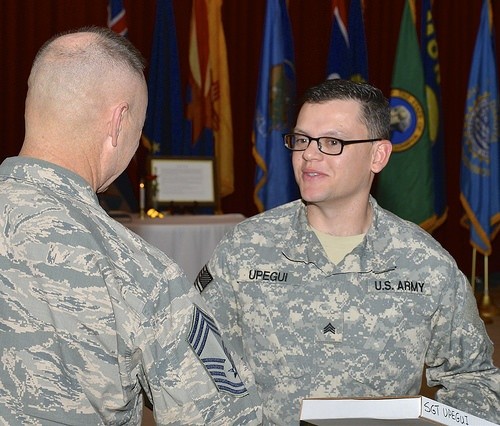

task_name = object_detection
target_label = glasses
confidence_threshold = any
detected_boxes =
[281,133,383,156]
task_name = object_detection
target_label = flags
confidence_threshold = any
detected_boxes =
[330,0,368,83]
[453,0,500,256]
[108,1,235,215]
[377,0,450,234]
[251,0,298,211]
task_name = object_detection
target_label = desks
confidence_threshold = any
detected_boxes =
[111,214,247,284]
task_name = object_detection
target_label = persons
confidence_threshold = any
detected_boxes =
[193,79,500,426]
[1,27,263,426]
